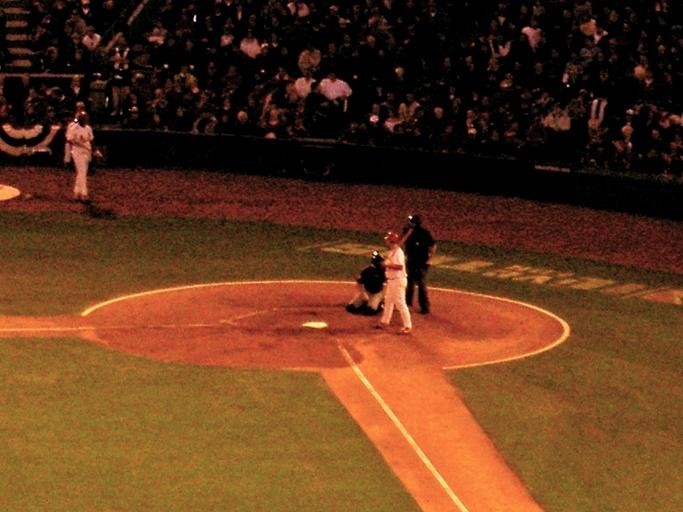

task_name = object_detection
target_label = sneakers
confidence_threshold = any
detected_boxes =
[395,330,409,335]
[81,194,91,204]
[73,192,81,201]
[372,322,389,331]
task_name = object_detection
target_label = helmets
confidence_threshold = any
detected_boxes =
[408,214,420,227]
[383,230,398,244]
[370,251,383,268]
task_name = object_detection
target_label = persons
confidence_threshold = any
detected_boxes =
[354,251,386,311]
[376,232,413,334]
[402,215,437,314]
[1,1,683,201]
